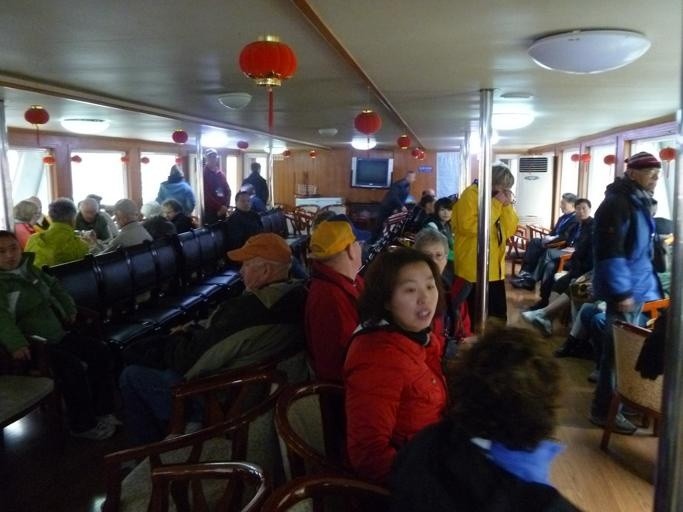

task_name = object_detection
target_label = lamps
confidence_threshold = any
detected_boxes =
[317,125,337,144]
[216,90,255,114]
[525,27,651,75]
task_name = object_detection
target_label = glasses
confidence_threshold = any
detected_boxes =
[423,251,448,260]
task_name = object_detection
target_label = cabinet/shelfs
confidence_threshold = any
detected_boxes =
[295,196,345,213]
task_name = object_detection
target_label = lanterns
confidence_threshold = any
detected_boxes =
[25,105,49,146]
[355,110,381,143]
[71,156,81,162]
[43,155,55,164]
[398,135,410,150]
[141,157,149,163]
[237,140,248,149]
[412,148,425,160]
[283,150,291,157]
[172,129,187,144]
[175,153,187,164]
[238,34,296,127]
[121,156,129,162]
[603,154,615,165]
[310,150,319,158]
[572,152,591,162]
[660,148,674,162]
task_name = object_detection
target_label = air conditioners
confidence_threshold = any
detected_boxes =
[515,152,556,233]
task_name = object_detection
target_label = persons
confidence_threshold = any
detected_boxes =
[379,170,458,249]
[158,164,196,213]
[117,232,307,449]
[589,151,667,434]
[508,193,607,383]
[0,230,123,442]
[384,325,581,512]
[202,149,269,248]
[307,220,473,480]
[13,192,193,269]
[452,165,520,332]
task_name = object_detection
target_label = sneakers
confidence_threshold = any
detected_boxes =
[590,411,636,434]
[588,369,601,383]
[521,309,546,323]
[70,420,116,441]
[535,316,553,336]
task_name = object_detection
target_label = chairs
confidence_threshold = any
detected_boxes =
[284,375,364,471]
[138,461,269,511]
[168,340,305,445]
[599,320,665,451]
[90,376,281,511]
[5,194,418,400]
[500,219,675,333]
[259,473,395,512]
[0,329,56,455]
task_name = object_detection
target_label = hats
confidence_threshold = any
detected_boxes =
[310,220,357,258]
[627,152,660,169]
[205,148,217,156]
[226,233,294,264]
[13,197,41,226]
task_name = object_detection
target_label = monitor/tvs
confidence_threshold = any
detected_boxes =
[350,156,394,189]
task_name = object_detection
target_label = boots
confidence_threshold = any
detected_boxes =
[552,335,577,357]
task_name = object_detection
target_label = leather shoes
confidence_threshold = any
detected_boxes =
[509,274,536,290]
[529,303,547,310]
